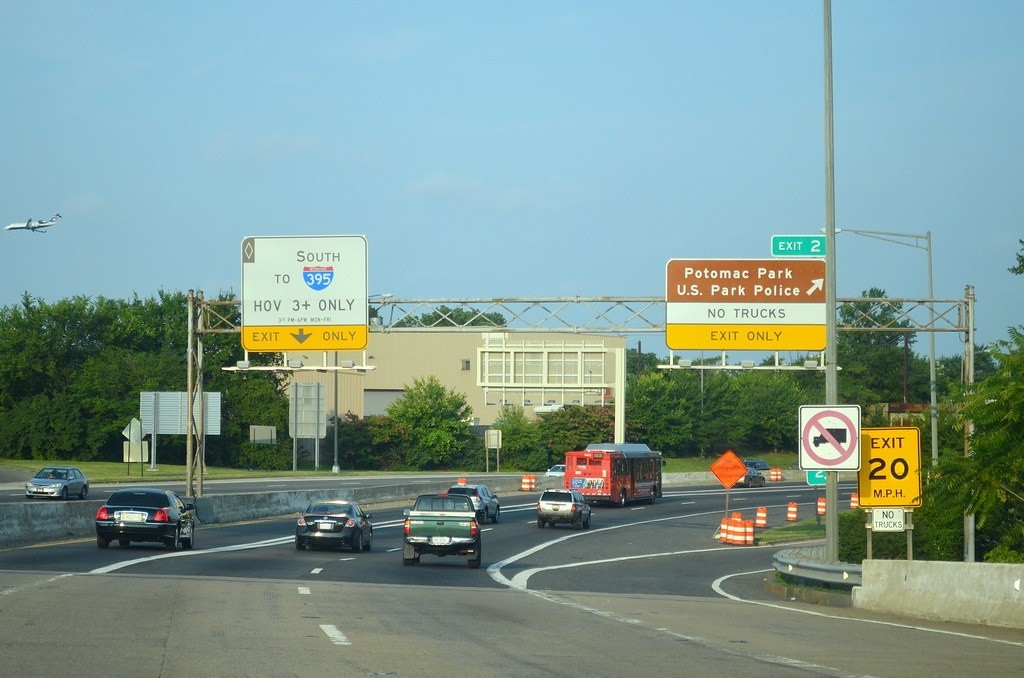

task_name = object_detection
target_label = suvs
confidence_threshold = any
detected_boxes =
[538,487,592,529]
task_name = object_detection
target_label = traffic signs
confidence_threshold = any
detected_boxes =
[239,236,367,352]
[668,257,828,349]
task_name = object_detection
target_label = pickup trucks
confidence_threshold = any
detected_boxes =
[402,492,483,568]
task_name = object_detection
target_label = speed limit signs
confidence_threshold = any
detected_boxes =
[858,426,922,509]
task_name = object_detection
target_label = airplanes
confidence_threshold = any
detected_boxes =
[4,212,63,233]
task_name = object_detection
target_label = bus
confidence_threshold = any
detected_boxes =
[566,442,666,509]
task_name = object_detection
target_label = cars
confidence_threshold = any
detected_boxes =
[732,468,767,487]
[543,465,567,477]
[296,500,373,553]
[445,483,499,526]
[24,466,90,501]
[742,458,769,471]
[93,489,196,552]
